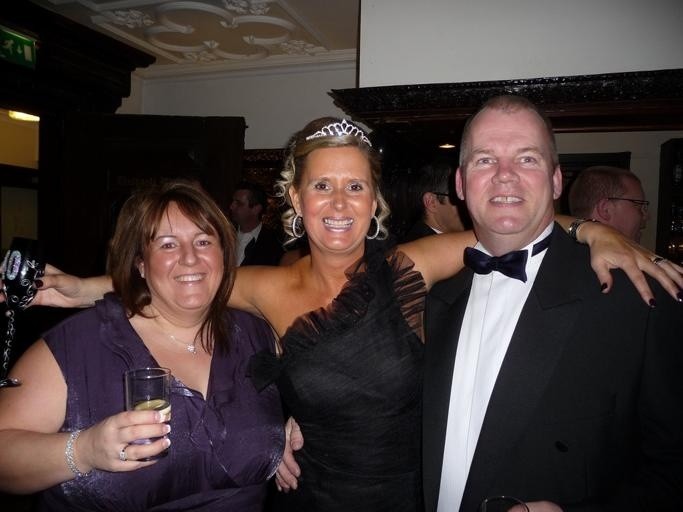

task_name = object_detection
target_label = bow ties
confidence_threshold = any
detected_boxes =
[463,232,553,283]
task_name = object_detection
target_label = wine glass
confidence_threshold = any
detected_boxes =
[0,235,51,387]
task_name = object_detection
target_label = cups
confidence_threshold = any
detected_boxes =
[123,367,172,463]
[481,495,530,512]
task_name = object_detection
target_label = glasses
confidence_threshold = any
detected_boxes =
[608,193,650,211]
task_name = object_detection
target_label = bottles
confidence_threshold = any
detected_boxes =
[666,220,682,261]
